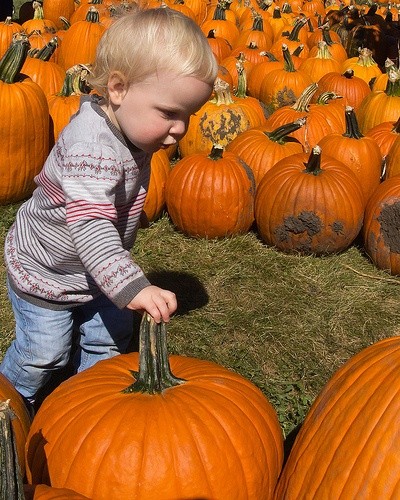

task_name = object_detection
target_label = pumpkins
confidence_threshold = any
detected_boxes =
[0,310,400,500]
[0,0,400,277]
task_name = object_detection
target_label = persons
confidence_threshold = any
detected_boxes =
[0,6,219,415]
